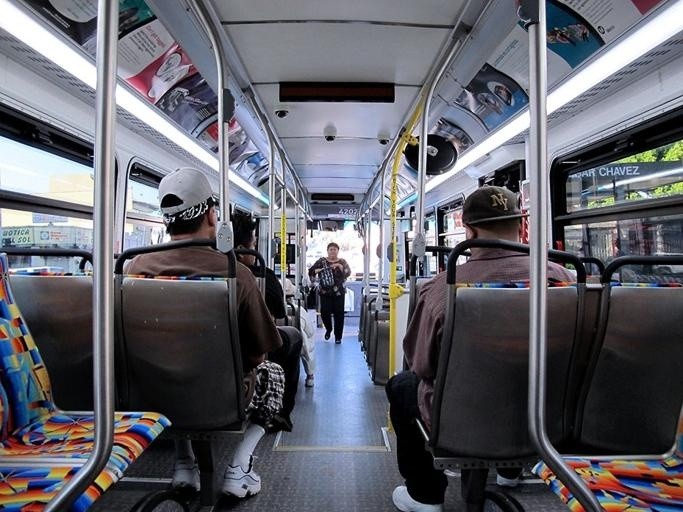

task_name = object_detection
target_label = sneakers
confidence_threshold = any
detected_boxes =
[391,484,444,512]
[335,339,342,344]
[324,332,331,340]
[305,378,315,387]
[221,453,263,500]
[169,454,202,494]
[495,473,523,488]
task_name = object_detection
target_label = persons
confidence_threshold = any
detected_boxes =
[306,243,351,346]
[386,184,572,510]
[271,279,315,390]
[121,169,287,499]
[219,208,301,438]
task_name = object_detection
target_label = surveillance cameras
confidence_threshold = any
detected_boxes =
[377,130,391,145]
[323,126,339,143]
[273,106,290,119]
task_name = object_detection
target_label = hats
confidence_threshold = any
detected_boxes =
[462,182,532,225]
[157,165,221,226]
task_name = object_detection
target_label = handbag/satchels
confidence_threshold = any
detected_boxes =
[318,267,336,290]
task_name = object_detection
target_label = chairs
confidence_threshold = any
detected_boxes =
[0,238,308,512]
[358,283,683,512]
